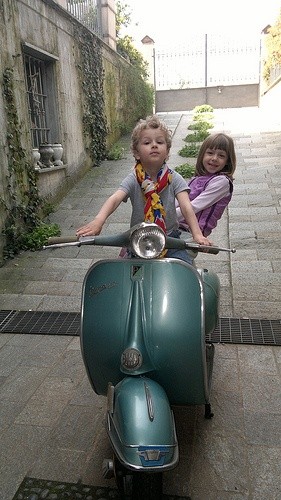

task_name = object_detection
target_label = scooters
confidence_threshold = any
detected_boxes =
[41,218,237,500]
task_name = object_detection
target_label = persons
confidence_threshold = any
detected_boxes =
[118,133,236,259]
[76,115,214,265]
